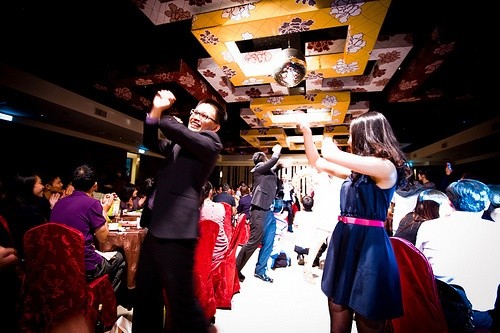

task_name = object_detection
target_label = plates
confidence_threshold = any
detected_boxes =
[126,212,142,217]
[119,221,136,227]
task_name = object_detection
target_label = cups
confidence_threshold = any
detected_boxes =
[108,223,119,230]
[136,217,142,229]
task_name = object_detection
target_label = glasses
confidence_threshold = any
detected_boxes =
[189,108,219,125]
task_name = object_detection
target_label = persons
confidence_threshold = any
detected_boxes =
[293,196,329,267]
[236,144,283,284]
[131,90,227,333]
[199,174,300,262]
[0,165,147,333]
[293,111,413,333]
[388,160,500,333]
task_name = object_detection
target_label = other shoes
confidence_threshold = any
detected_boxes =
[254,272,274,283]
[238,271,245,282]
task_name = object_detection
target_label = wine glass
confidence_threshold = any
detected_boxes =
[107,210,121,223]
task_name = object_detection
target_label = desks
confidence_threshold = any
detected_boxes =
[100,216,148,289]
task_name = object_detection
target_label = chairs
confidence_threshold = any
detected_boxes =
[16,202,261,333]
[390,236,447,333]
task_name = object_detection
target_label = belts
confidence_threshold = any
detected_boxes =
[337,215,385,228]
